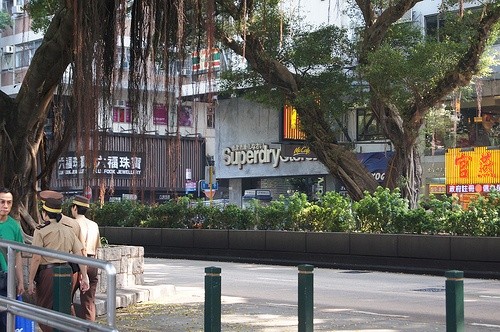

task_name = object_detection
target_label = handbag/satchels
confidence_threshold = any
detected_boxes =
[15,295,35,332]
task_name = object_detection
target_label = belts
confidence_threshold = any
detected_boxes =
[39,263,66,270]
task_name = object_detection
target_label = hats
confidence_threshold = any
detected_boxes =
[39,191,63,204]
[42,197,63,214]
[71,195,89,208]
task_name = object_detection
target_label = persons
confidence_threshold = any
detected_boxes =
[70,195,101,322]
[0,187,26,331]
[37,190,90,284]
[28,198,90,332]
